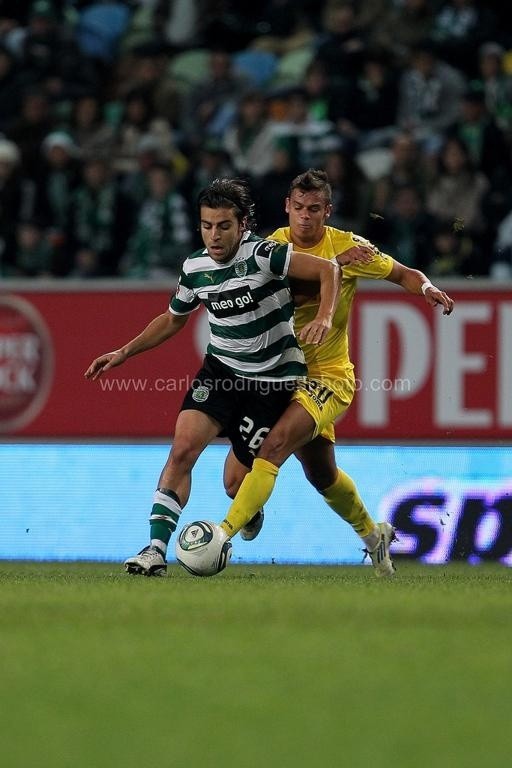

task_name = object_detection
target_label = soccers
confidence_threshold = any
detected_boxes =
[174,519,233,576]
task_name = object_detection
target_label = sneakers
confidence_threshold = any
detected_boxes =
[124,546,168,578]
[365,522,399,578]
[239,507,264,541]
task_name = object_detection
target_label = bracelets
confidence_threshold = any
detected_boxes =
[421,282,433,295]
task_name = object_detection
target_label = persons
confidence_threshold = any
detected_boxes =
[214,168,455,579]
[82,175,343,576]
